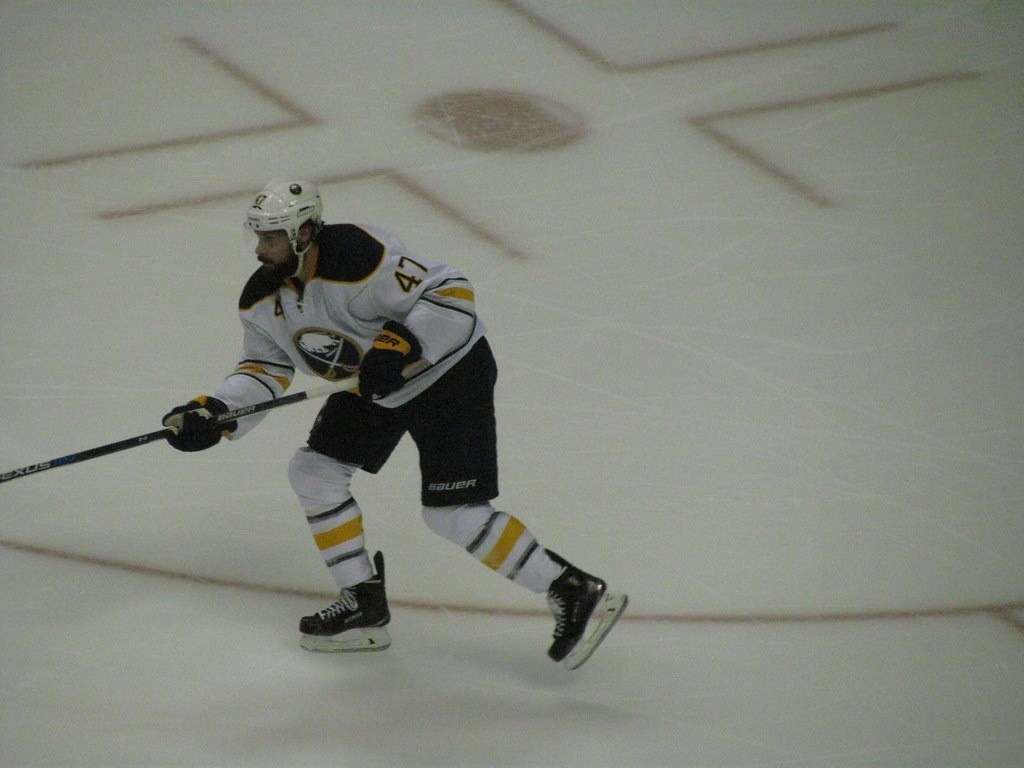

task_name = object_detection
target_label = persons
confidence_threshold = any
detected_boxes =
[162,177,608,663]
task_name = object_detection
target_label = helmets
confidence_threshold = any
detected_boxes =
[243,175,323,241]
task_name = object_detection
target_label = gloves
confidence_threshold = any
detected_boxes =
[163,395,238,452]
[358,320,423,401]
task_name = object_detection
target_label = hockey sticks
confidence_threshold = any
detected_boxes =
[0,376,360,484]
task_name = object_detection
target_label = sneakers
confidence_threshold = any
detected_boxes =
[544,549,628,670]
[300,550,393,652]
